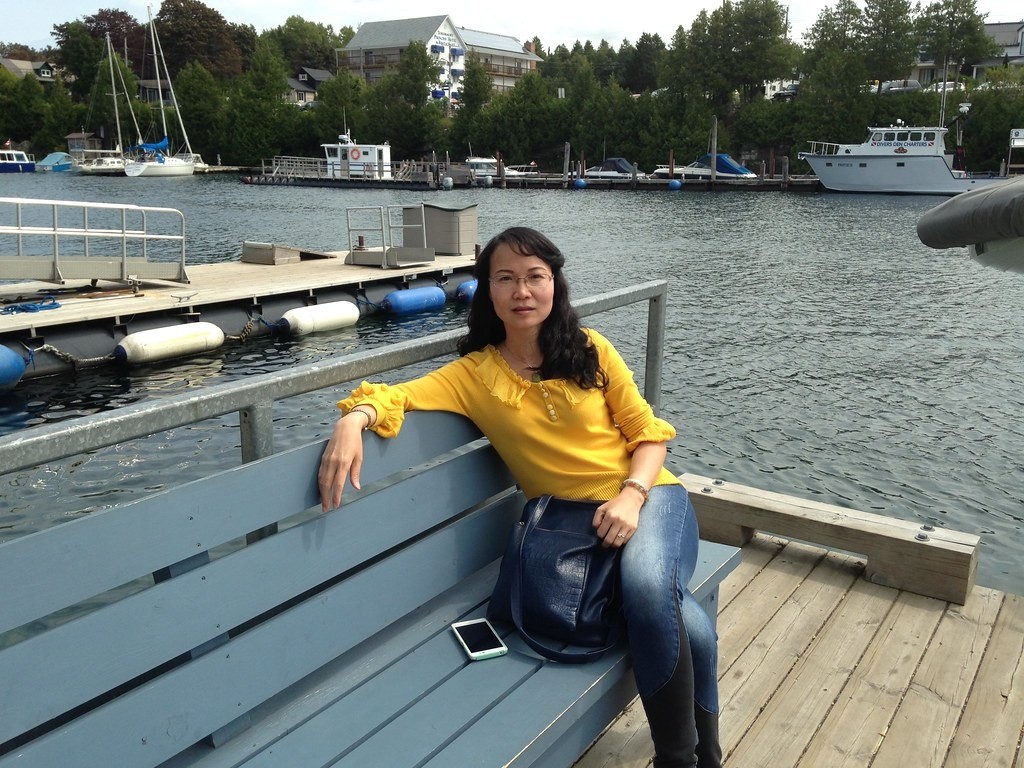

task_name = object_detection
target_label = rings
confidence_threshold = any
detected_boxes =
[618,533,625,538]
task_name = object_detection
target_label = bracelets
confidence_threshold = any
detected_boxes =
[619,479,650,503]
[346,409,371,432]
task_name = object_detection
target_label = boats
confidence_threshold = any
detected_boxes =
[234,140,443,192]
[72,155,130,174]
[568,158,647,178]
[124,153,196,177]
[655,149,756,179]
[798,127,1006,195]
[465,156,529,176]
[34,150,73,171]
[0,150,35,173]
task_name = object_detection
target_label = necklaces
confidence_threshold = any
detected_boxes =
[502,344,540,382]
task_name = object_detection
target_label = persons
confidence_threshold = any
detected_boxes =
[317,226,722,768]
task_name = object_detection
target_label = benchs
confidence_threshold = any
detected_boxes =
[0,404,747,768]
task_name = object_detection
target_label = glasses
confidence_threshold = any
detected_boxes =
[488,272,554,289]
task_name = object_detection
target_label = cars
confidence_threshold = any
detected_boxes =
[631,77,1019,99]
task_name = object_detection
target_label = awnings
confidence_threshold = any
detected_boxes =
[452,92,461,98]
[451,69,464,76]
[431,45,444,53]
[451,48,464,55]
[440,68,445,74]
[432,91,445,98]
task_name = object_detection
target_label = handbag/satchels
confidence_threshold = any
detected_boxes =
[486,494,620,664]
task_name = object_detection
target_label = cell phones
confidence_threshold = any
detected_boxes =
[451,617,508,661]
